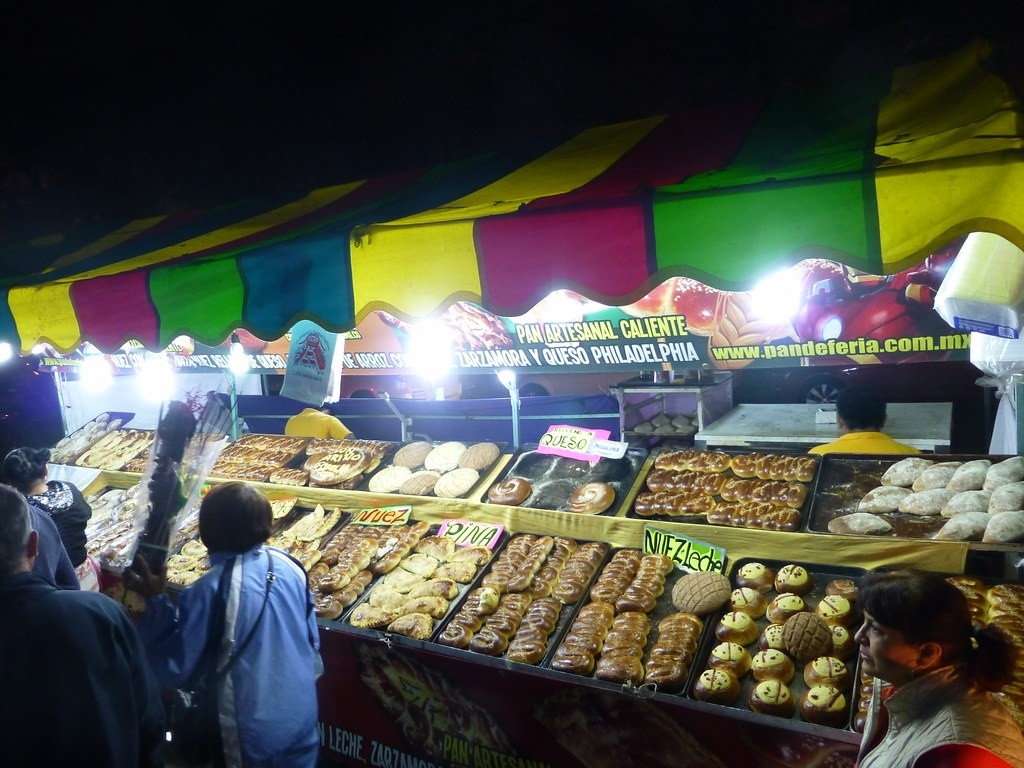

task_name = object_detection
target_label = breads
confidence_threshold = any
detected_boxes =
[566,480,615,515]
[488,477,532,506]
[77,479,340,587]
[180,431,498,499]
[852,574,1024,734]
[549,549,730,691]
[45,412,158,473]
[631,415,698,434]
[634,449,818,531]
[693,561,863,728]
[304,519,608,666]
[828,456,1024,543]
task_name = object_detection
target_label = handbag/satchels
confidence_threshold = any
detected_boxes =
[171,689,218,768]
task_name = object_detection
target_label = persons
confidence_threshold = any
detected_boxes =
[0,483,158,768]
[808,377,922,454]
[28,504,80,590]
[854,563,1024,768]
[136,480,322,767]
[2,447,100,596]
[284,404,355,439]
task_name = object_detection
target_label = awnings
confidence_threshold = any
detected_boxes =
[0,37,1023,418]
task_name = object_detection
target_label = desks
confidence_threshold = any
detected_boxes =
[694,402,954,454]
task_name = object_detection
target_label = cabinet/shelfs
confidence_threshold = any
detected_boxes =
[610,372,733,451]
